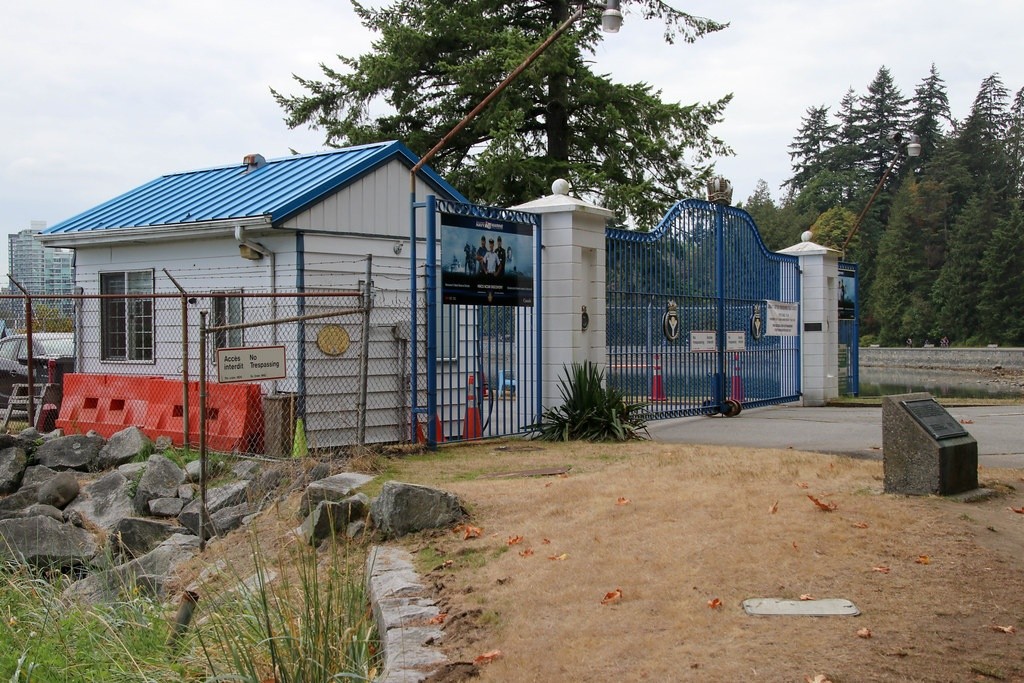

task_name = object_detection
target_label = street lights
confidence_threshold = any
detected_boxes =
[842,134,921,262]
[409,0,624,450]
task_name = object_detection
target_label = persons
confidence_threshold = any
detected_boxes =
[838,279,844,307]
[907,336,949,348]
[463,236,517,278]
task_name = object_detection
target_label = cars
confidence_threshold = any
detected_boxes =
[0,333,75,410]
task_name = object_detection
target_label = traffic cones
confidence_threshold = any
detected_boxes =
[292,416,308,460]
[732,352,746,402]
[416,412,447,444]
[462,376,482,442]
[648,354,669,401]
